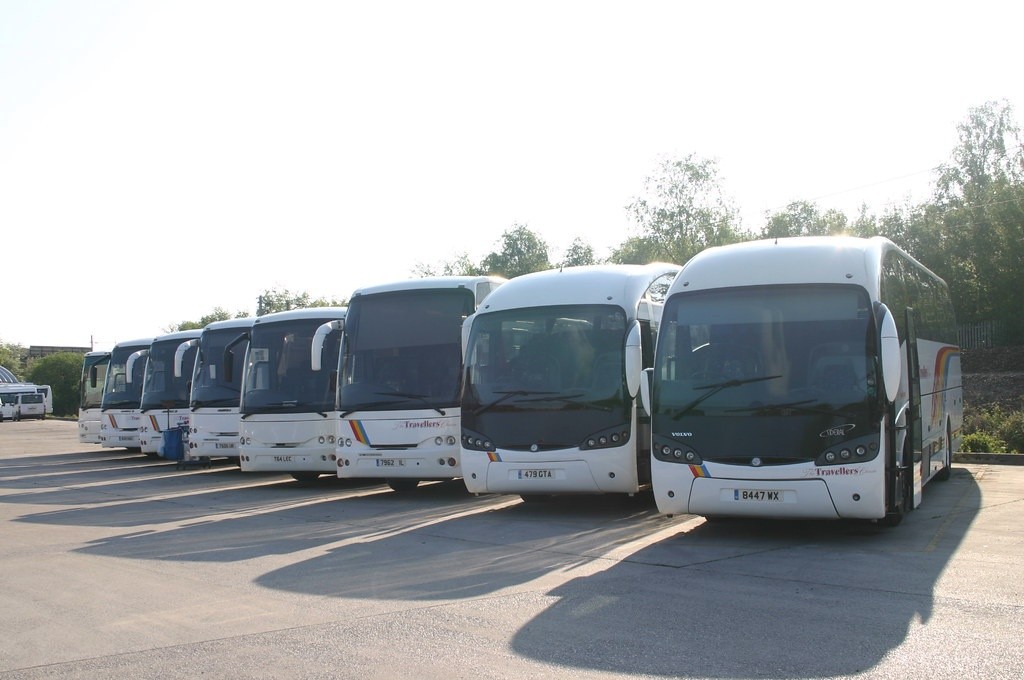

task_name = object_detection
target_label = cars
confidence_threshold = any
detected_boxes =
[0,397,5,422]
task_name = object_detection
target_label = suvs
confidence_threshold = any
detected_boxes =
[10,393,46,421]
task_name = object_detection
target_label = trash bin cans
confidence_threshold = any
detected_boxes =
[162,429,183,459]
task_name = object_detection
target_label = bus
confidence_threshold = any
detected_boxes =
[89,336,156,452]
[126,328,203,458]
[459,262,683,508]
[638,236,965,529]
[174,316,287,468]
[310,275,508,497]
[78,351,111,446]
[223,306,349,485]
[0,382,54,417]
